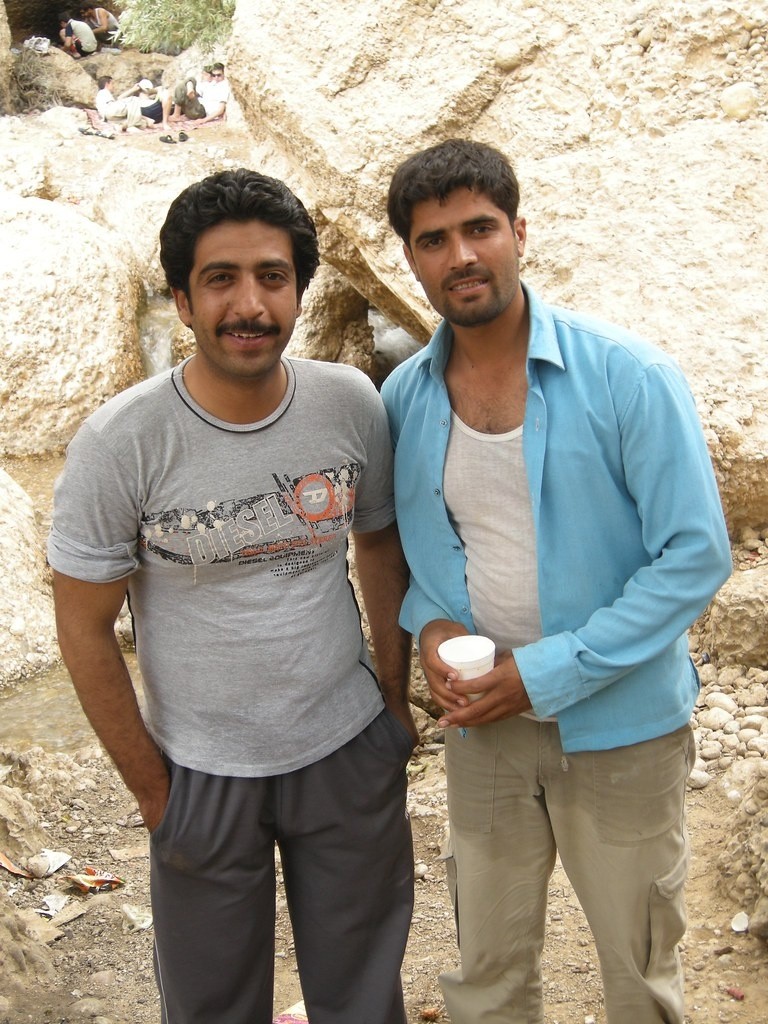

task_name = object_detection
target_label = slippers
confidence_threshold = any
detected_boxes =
[179,131,188,142]
[160,135,177,143]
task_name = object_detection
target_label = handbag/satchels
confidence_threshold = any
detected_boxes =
[24,36,50,56]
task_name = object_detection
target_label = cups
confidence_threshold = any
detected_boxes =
[438,635,495,704]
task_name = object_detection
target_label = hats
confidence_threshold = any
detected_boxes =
[139,78,153,92]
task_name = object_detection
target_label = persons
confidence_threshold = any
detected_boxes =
[379,138,734,1024]
[46,169,418,1024]
[96,63,232,132]
[58,13,98,58]
[80,4,123,52]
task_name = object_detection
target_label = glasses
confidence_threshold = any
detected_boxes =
[212,74,222,78]
